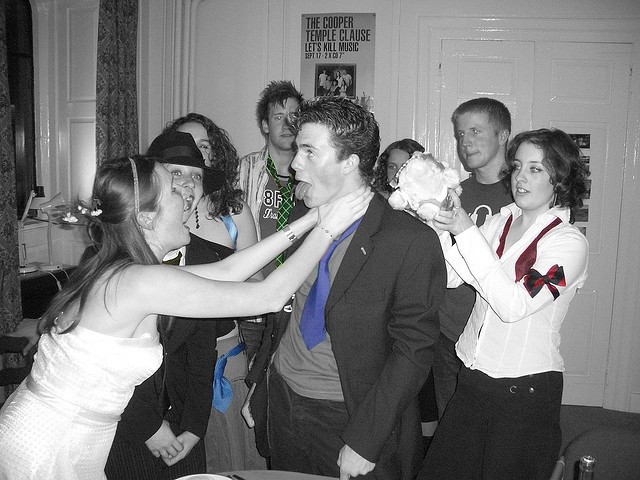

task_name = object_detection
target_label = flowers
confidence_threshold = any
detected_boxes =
[76,198,102,217]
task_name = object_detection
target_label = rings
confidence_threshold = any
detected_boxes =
[452,212,458,219]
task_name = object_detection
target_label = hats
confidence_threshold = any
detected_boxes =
[144,130,227,199]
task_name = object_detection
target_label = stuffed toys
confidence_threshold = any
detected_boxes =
[387,150,463,237]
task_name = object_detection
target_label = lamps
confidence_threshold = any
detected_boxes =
[18,185,47,274]
[38,190,66,271]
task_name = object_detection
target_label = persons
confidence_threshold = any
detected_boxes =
[426,128,590,479]
[431,96,513,421]
[4,112,268,473]
[240,95,448,480]
[318,68,352,95]
[103,131,218,480]
[372,139,426,200]
[234,80,305,370]
[0,156,374,480]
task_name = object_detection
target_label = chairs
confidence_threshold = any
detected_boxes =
[550,425,640,480]
[0,336,37,409]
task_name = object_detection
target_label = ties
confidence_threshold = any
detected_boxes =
[298,214,363,352]
[495,214,563,284]
[266,150,296,269]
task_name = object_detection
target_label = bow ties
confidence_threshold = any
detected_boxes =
[522,263,566,300]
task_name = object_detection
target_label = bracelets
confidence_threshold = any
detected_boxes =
[281,224,298,243]
[314,224,335,242]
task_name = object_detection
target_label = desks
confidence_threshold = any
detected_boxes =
[21,265,76,318]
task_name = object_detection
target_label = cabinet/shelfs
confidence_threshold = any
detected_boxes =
[18,220,48,266]
